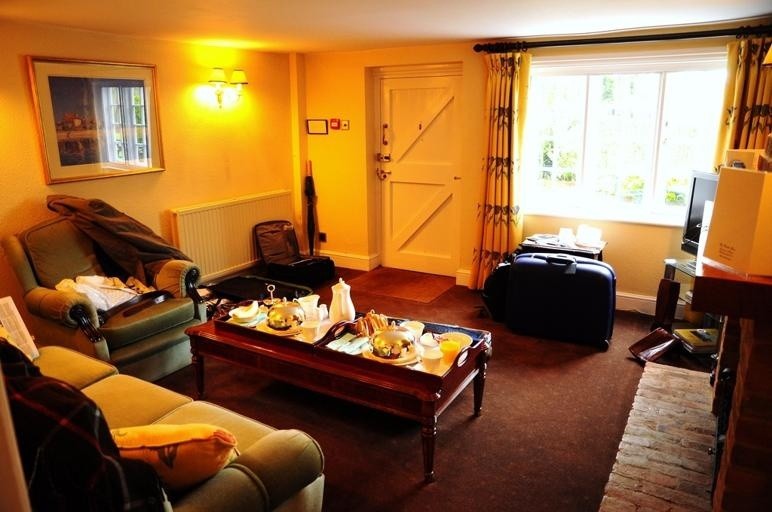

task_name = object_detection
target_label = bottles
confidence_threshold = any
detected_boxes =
[319,304,328,319]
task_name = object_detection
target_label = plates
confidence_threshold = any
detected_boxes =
[416,347,444,360]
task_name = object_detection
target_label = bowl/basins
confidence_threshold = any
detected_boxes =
[399,321,425,340]
[263,298,281,310]
[228,307,261,323]
[440,333,473,356]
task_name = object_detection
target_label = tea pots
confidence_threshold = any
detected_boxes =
[329,278,356,324]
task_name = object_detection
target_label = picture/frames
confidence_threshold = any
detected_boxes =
[25,53,166,185]
[307,119,328,135]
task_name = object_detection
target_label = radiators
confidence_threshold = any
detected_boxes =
[171,190,292,286]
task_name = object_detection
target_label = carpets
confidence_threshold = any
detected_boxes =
[350,266,458,303]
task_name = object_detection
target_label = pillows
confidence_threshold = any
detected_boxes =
[20,217,101,286]
[110,424,240,495]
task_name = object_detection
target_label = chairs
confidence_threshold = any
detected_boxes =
[4,212,207,383]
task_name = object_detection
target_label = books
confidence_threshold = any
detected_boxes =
[673,328,721,355]
[1,296,40,363]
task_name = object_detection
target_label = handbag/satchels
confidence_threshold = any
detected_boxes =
[481,259,512,323]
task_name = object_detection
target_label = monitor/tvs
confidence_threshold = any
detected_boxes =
[679,173,720,269]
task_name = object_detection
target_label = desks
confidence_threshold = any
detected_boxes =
[690,200,772,511]
[519,234,607,260]
[650,258,725,369]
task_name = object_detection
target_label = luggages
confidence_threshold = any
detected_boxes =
[254,220,337,289]
[503,251,616,352]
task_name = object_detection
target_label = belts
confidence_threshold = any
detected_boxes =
[97,290,170,324]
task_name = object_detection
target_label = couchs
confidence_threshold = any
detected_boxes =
[0,346,326,512]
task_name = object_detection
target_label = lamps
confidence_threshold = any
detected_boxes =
[208,68,248,101]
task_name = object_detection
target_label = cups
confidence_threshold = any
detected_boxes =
[420,333,440,352]
[292,294,320,315]
[301,321,320,339]
